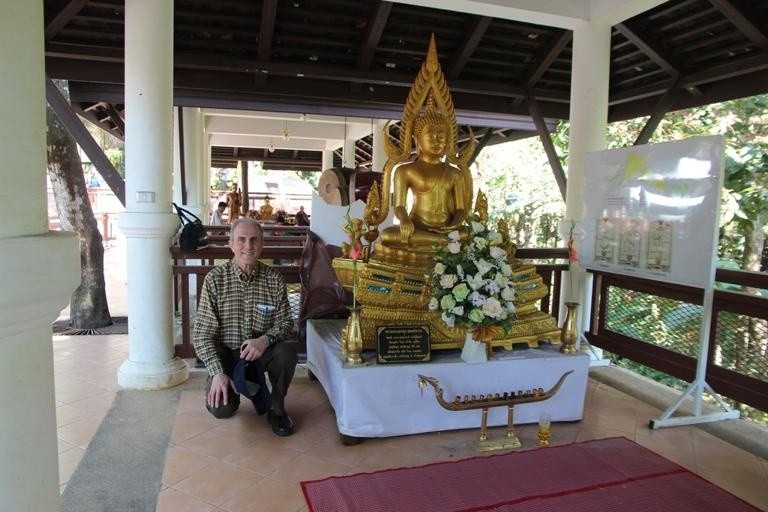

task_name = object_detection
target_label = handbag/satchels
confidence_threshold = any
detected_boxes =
[172,203,208,252]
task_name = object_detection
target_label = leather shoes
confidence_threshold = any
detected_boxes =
[267,410,294,436]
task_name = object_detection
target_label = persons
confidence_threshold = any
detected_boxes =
[261,195,273,219]
[378,92,475,248]
[226,182,242,225]
[295,205,310,226]
[210,201,229,226]
[192,217,299,438]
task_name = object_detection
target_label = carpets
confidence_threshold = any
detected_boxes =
[300,436,765,512]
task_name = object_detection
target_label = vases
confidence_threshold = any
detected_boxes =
[460,328,489,363]
[560,302,581,354]
[345,304,364,364]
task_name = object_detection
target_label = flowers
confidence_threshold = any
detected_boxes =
[343,202,367,308]
[425,213,522,333]
[566,218,578,301]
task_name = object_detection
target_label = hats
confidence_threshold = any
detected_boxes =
[233,358,273,416]
